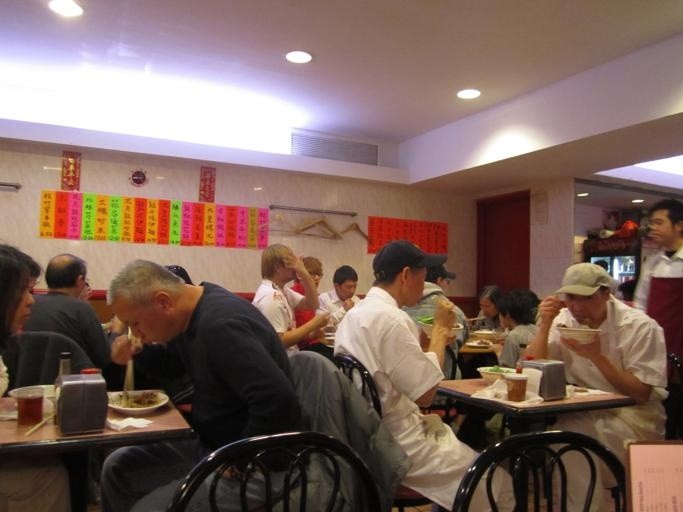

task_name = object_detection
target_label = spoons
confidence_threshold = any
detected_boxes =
[469,316,488,322]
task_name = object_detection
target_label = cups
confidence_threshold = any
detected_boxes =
[504,373,529,402]
[16,386,45,427]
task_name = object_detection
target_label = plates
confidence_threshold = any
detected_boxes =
[0,397,54,422]
[106,390,170,414]
[8,384,57,398]
[463,339,492,348]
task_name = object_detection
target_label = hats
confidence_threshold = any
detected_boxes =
[373,239,449,280]
[553,262,615,297]
[424,264,457,282]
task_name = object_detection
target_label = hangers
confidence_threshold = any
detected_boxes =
[254,206,372,243]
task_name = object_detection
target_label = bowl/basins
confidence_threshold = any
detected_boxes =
[415,316,465,341]
[557,326,602,344]
[476,366,518,385]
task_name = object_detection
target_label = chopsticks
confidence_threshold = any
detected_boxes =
[319,307,339,322]
[123,324,134,392]
[24,416,52,440]
[431,294,463,315]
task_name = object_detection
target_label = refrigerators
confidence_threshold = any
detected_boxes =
[582,233,663,301]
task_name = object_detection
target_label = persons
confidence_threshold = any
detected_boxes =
[2,243,70,510]
[289,257,334,361]
[515,262,666,511]
[101,259,309,511]
[632,199,682,440]
[20,254,196,504]
[468,285,541,369]
[315,265,361,349]
[401,264,470,424]
[334,242,517,510]
[251,243,331,358]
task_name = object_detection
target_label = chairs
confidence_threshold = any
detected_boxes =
[0,332,644,512]
[663,353,683,440]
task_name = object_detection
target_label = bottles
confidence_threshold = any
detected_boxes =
[516,355,535,375]
[52,352,73,427]
